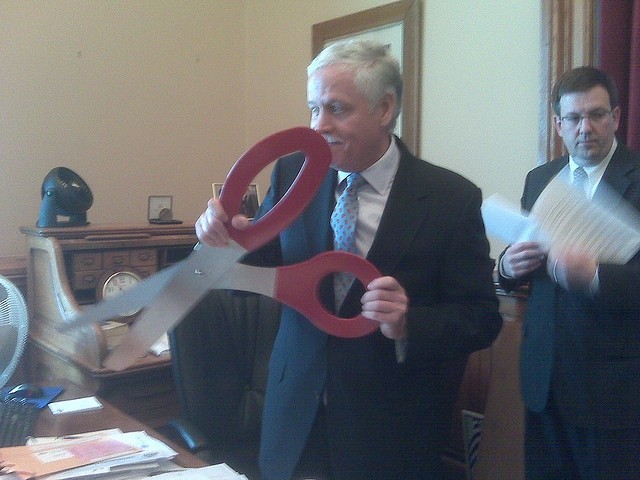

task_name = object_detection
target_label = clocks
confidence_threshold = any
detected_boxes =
[94,264,152,322]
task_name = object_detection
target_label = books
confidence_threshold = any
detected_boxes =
[97,322,131,355]
[6,427,187,480]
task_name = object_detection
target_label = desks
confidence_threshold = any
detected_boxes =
[0,378,211,480]
[19,222,199,430]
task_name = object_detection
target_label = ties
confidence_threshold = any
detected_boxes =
[330,173,368,315]
[573,168,587,199]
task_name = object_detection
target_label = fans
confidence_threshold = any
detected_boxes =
[0,274,31,392]
[36,166,93,228]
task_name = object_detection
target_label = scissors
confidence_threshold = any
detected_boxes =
[58,127,383,373]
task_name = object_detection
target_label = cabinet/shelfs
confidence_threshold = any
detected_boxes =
[443,290,526,480]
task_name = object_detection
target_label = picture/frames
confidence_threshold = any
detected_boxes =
[461,408,485,479]
[212,183,260,221]
[311,0,422,159]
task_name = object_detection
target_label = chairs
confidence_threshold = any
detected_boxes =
[157,246,265,480]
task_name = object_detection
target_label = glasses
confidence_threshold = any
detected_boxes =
[562,112,603,124]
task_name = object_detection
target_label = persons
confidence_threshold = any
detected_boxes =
[195,41,502,480]
[497,67,640,480]
